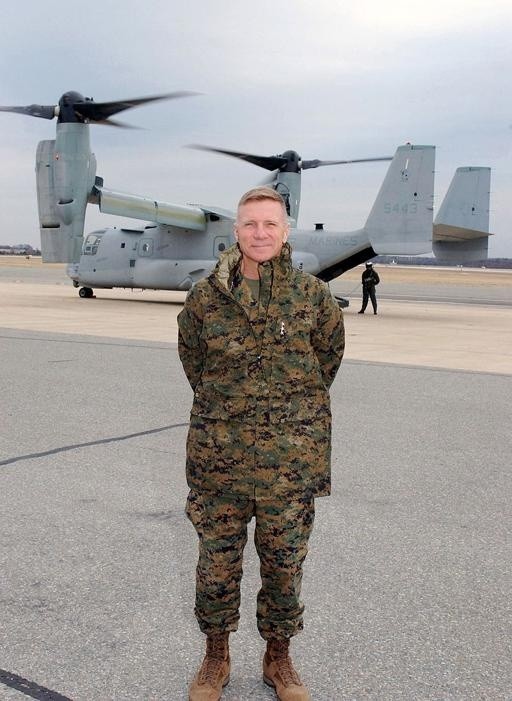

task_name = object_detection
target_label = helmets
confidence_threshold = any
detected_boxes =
[366,262,372,268]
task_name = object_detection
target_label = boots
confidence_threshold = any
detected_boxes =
[190,632,230,700]
[262,639,310,700]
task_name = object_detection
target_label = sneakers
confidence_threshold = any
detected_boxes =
[374,312,377,314]
[358,311,364,313]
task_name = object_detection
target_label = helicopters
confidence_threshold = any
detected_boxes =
[1,83,498,312]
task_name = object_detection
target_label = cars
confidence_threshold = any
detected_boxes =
[1,242,40,255]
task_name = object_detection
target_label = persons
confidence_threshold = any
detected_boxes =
[355,260,382,314]
[176,187,346,701]
